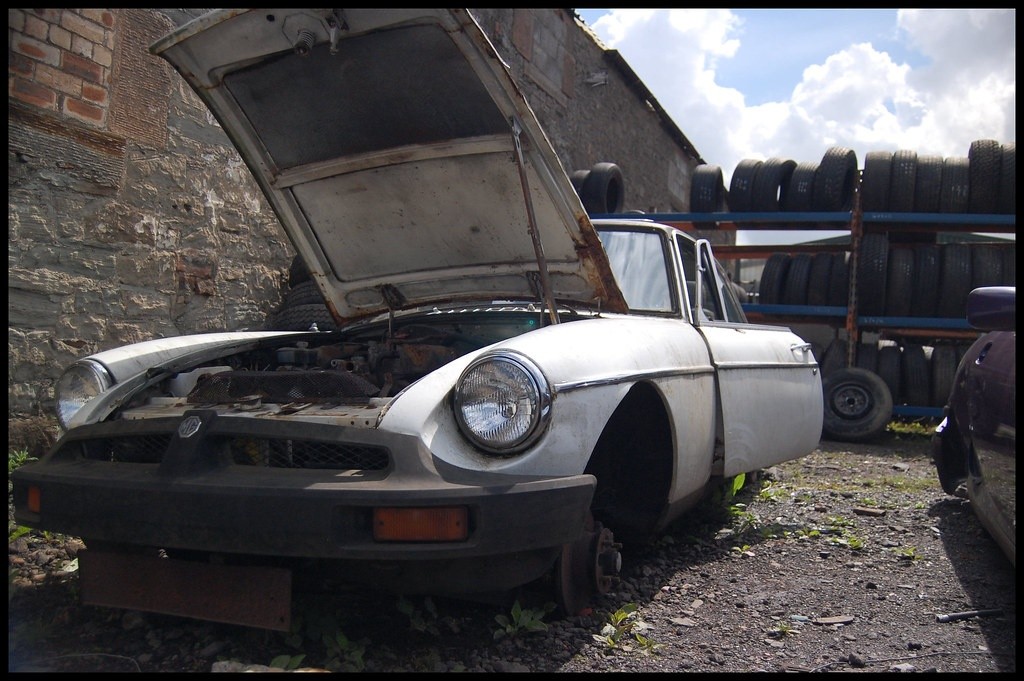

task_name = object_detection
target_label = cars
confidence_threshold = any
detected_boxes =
[10,6,824,620]
[933,285,1016,568]
